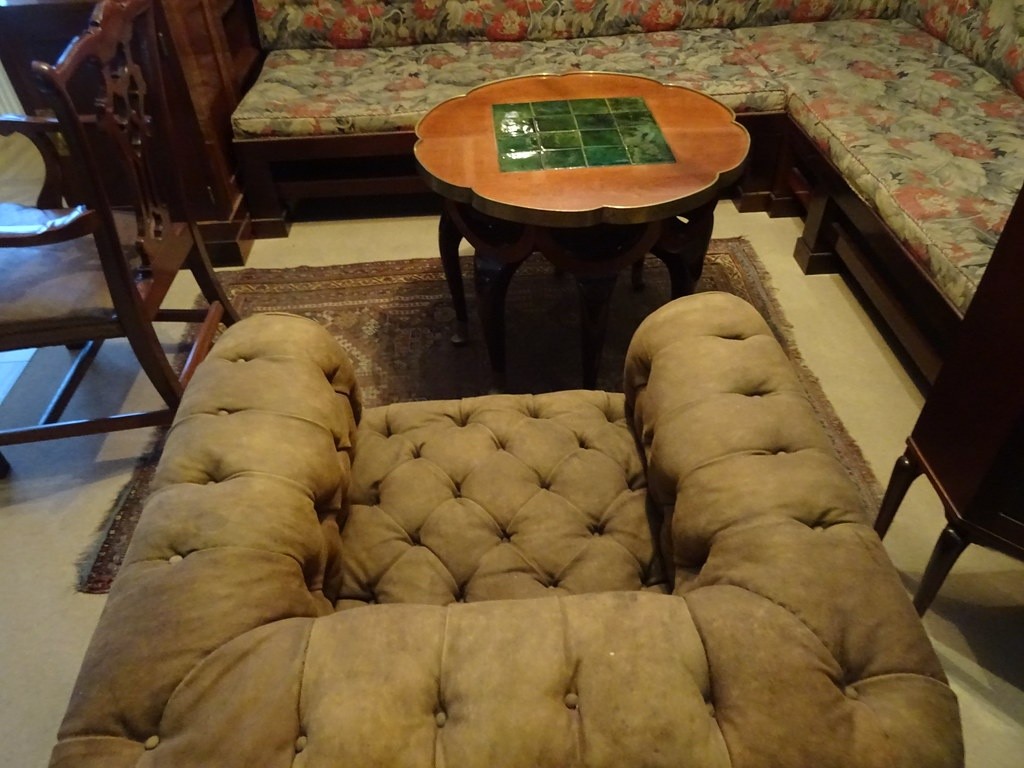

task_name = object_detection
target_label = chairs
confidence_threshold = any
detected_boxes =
[1,1,241,481]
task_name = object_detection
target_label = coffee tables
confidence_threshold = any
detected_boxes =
[413,68,751,396]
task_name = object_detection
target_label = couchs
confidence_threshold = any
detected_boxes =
[227,2,1024,396]
[48,290,971,768]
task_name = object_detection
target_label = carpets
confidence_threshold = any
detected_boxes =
[69,233,887,595]
